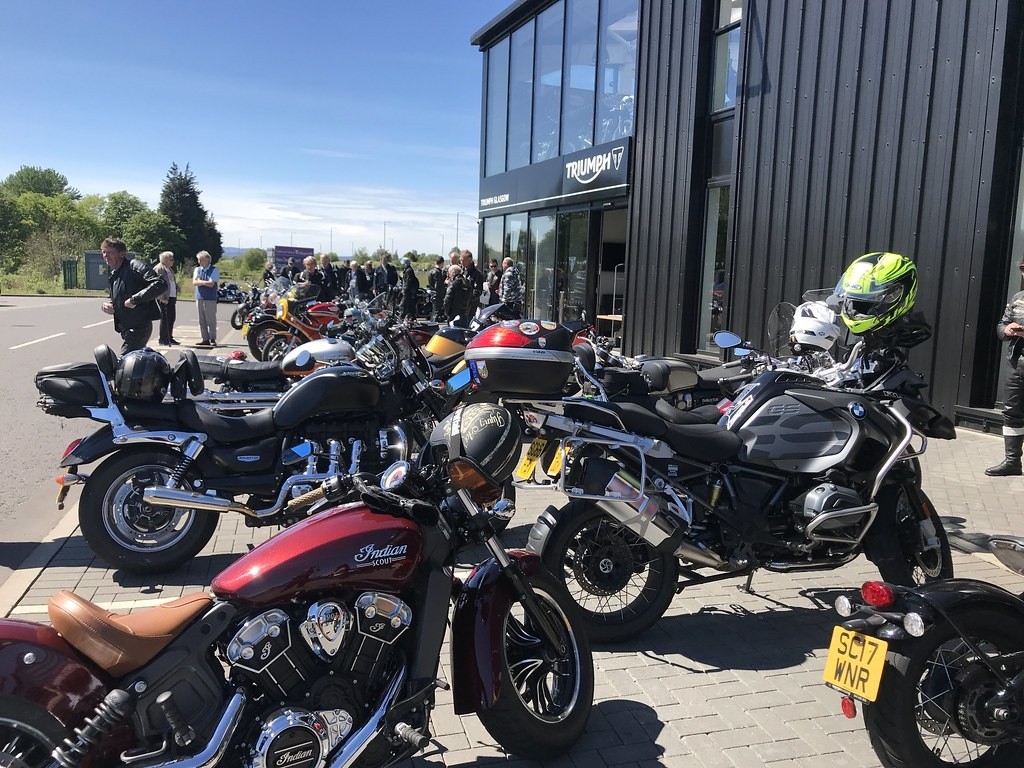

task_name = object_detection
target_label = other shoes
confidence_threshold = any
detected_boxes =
[196,341,210,344]
[160,338,179,345]
[210,340,216,346]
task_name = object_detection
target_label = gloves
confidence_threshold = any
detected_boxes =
[352,475,439,528]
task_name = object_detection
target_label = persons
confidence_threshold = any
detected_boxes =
[279,254,398,309]
[153,251,180,345]
[428,251,521,329]
[517,262,564,320]
[193,251,219,345]
[100,238,169,361]
[985,257,1024,477]
[398,257,416,321]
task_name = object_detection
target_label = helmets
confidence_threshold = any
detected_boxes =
[789,301,840,351]
[113,347,169,405]
[430,401,523,493]
[839,253,917,335]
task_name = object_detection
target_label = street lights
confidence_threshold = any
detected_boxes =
[389,237,394,253]
[439,234,444,258]
[291,232,295,248]
[330,227,336,253]
[456,211,466,248]
[384,221,391,252]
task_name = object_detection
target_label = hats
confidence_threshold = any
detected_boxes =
[288,257,295,264]
[400,257,411,266]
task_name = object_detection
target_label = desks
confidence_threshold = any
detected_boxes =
[597,314,622,321]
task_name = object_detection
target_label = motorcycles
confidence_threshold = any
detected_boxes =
[0,435,595,767]
[824,534,1024,768]
[32,270,957,648]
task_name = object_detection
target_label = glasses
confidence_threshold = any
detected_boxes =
[490,265,497,268]
[445,455,502,503]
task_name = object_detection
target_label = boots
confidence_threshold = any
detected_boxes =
[985,426,1024,477]
